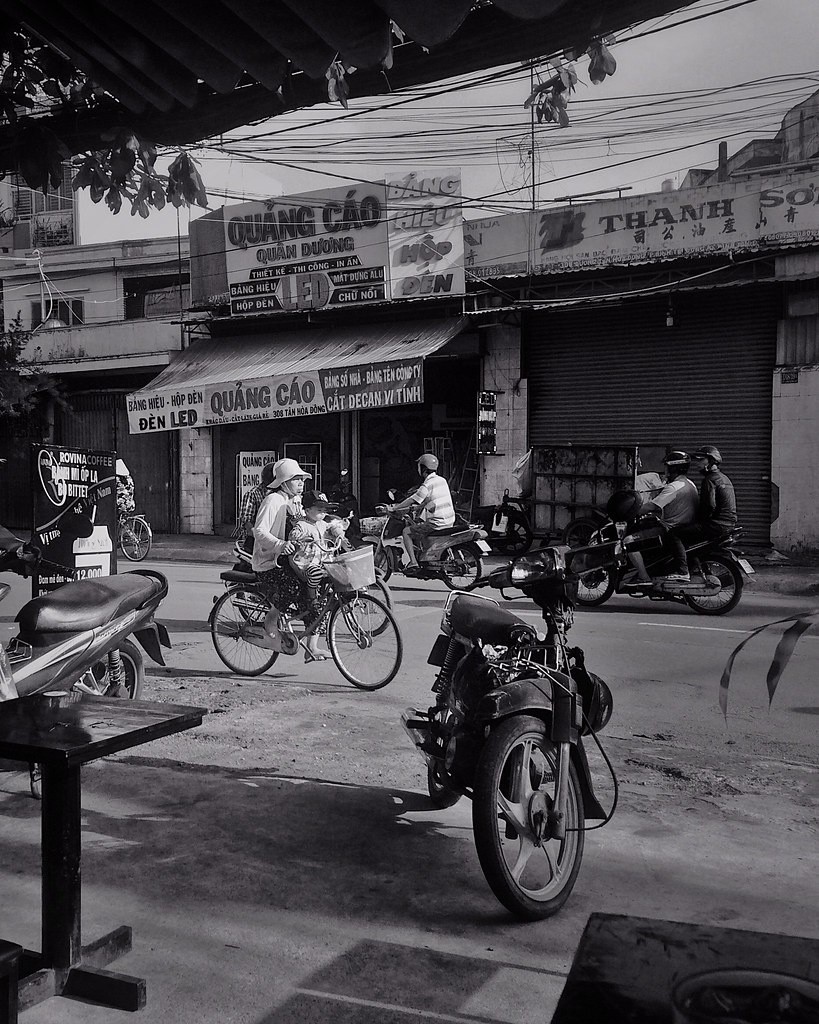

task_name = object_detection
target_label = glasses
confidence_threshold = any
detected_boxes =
[695,456,706,462]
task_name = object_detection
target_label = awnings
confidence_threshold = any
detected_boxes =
[123,316,467,435]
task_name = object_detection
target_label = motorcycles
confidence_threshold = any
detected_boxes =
[314,467,360,533]
[357,491,493,595]
[561,488,757,611]
[397,534,621,925]
[228,513,394,637]
[0,525,171,700]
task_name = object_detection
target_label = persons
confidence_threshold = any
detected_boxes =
[623,445,737,585]
[239,460,357,664]
[115,457,137,515]
[387,454,456,572]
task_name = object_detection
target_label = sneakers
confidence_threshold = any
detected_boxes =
[665,572,691,582]
[624,578,653,586]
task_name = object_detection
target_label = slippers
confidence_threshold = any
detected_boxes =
[264,614,279,640]
[402,559,419,571]
[304,649,333,663]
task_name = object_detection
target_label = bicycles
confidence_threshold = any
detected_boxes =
[206,534,404,692]
[116,510,153,564]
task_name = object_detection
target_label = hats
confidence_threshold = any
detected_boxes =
[266,458,312,488]
[116,459,130,476]
[301,490,329,508]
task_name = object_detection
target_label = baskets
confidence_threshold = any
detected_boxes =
[321,544,376,593]
[359,517,388,536]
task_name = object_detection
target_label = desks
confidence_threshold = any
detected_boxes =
[0,694,209,1023]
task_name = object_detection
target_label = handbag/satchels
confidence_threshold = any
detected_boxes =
[492,513,508,532]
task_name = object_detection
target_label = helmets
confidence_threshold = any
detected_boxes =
[660,451,691,475]
[694,445,722,465]
[607,490,642,522]
[568,662,613,737]
[414,454,439,470]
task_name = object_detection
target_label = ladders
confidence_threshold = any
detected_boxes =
[454,425,481,524]
